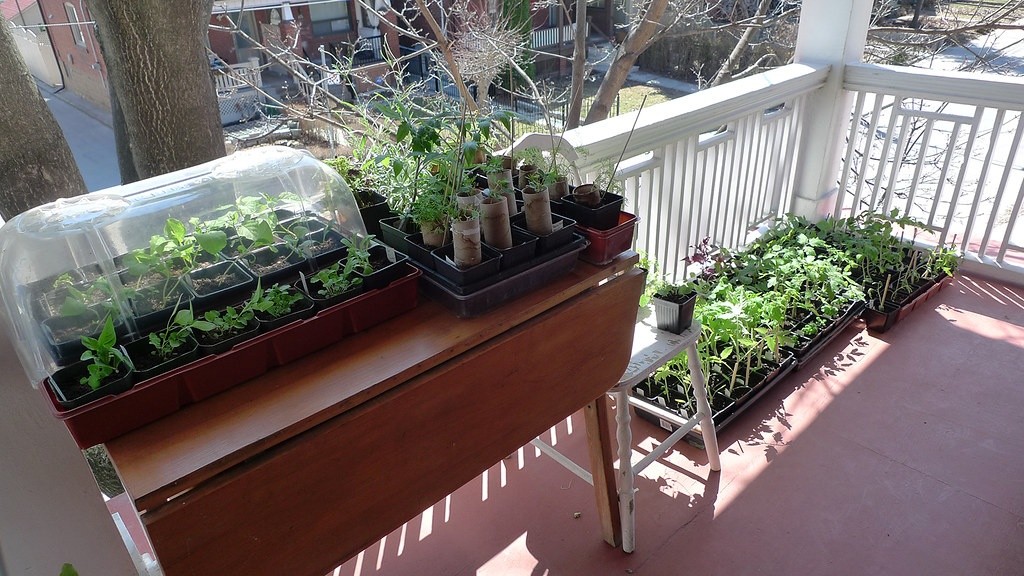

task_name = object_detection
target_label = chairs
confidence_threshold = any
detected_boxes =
[502,132,721,553]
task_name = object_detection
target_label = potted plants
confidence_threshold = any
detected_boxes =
[25,100,965,444]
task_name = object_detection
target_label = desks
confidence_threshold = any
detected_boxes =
[104,249,647,576]
